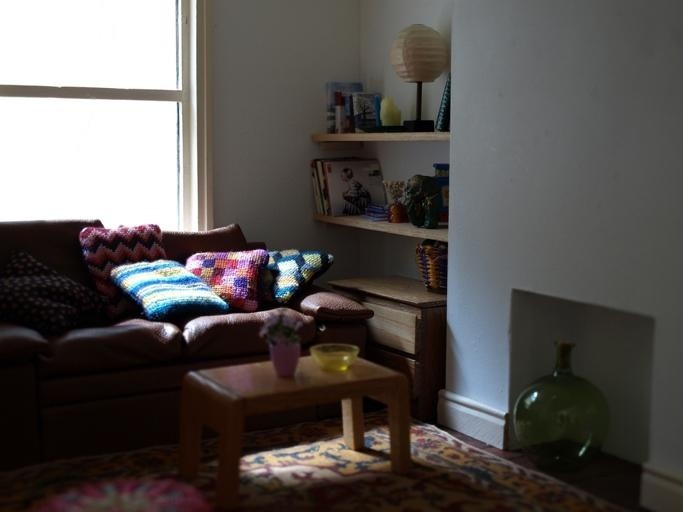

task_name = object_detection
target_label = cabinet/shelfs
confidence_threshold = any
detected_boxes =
[325,273,446,422]
[310,128,450,245]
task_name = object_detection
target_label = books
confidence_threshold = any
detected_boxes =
[325,81,381,133]
[310,157,388,217]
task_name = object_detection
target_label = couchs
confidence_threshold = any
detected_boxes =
[0,219,374,470]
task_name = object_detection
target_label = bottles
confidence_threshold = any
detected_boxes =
[430,163,451,222]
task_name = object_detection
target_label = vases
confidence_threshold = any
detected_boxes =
[265,337,300,381]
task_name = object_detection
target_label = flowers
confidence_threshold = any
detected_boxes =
[253,312,305,346]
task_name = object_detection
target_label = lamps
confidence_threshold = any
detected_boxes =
[388,21,450,131]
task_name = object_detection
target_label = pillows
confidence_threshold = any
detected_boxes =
[107,254,234,322]
[182,246,269,316]
[252,246,334,307]
[76,223,168,323]
[1,243,112,340]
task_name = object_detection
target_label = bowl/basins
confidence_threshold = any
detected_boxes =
[309,342,360,370]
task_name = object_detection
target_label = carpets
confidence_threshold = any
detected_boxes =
[0,408,639,511]
